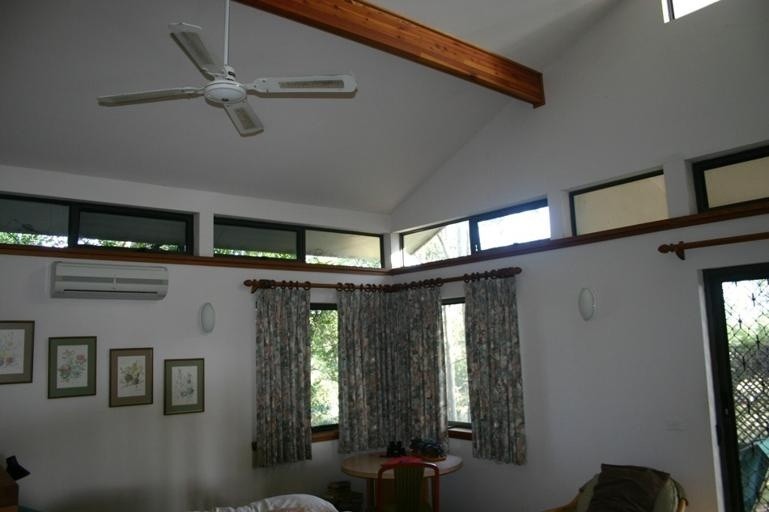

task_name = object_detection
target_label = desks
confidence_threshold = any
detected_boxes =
[342,452,463,511]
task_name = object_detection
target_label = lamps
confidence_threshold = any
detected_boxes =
[578,288,594,320]
[200,303,216,332]
[5,455,31,481]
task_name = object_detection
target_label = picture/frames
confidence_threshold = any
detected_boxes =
[163,357,206,415]
[47,336,97,399]
[108,347,154,407]
[0,320,35,384]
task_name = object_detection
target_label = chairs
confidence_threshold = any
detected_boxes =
[376,459,442,511]
[543,464,690,512]
[738,435,769,511]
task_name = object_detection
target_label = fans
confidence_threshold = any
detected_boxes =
[96,0,357,138]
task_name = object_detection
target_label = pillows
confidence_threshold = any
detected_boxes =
[216,493,339,511]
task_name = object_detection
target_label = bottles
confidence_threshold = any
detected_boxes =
[385,441,401,455]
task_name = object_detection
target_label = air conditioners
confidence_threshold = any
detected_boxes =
[49,262,170,301]
